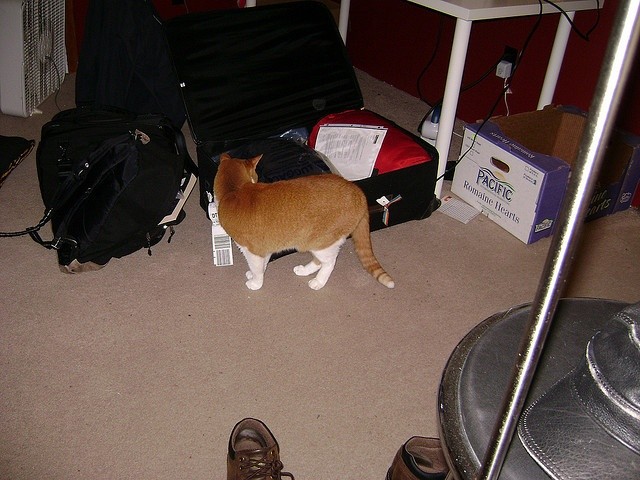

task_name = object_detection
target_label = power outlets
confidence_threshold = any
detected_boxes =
[503,46,518,74]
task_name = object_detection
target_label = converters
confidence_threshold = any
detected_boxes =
[495,61,512,79]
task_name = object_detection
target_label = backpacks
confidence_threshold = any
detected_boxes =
[36,105,198,275]
[75,3,186,127]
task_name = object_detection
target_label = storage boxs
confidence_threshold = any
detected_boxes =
[451,104,640,245]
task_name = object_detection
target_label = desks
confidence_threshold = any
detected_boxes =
[339,0,605,200]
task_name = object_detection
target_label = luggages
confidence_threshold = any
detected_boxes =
[160,0,442,262]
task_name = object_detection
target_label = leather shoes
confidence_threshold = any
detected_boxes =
[387,435,450,478]
[226,418,295,480]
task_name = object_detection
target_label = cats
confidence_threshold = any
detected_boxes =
[213,152,395,291]
